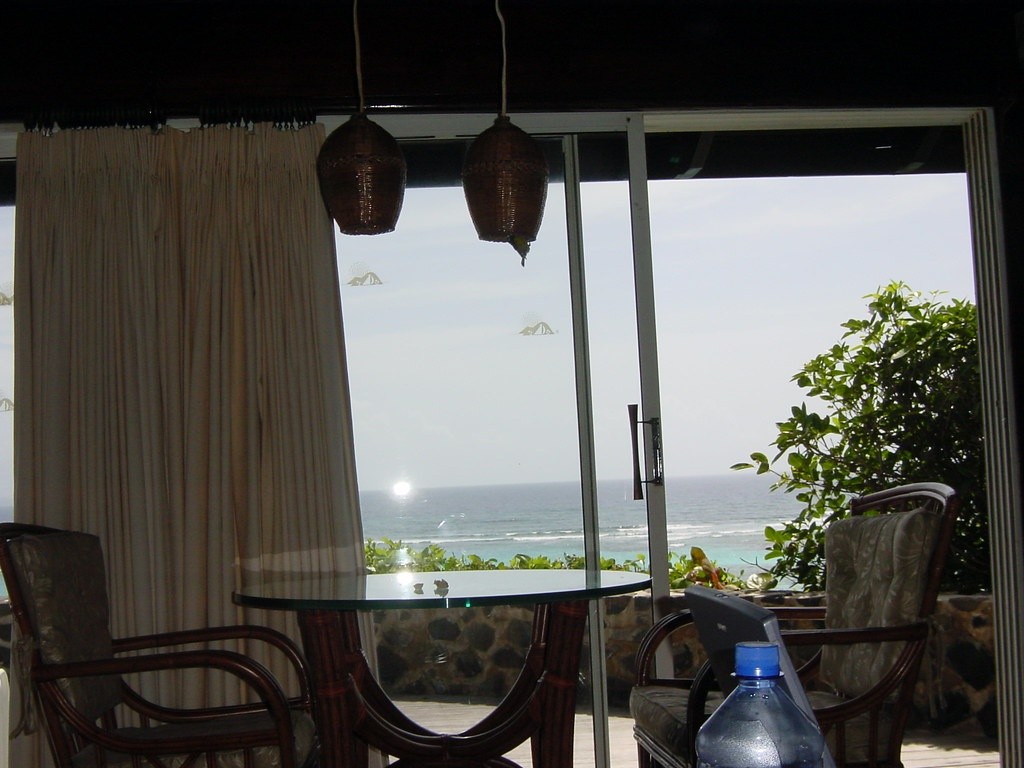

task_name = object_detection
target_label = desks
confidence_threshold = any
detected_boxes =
[232,568,651,767]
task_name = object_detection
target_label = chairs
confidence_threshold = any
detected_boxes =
[629,481,954,767]
[0,522,324,767]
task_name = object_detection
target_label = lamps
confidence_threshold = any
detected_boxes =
[316,0,406,236]
[461,0,549,242]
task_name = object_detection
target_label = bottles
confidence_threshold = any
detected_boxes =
[696,641,824,768]
